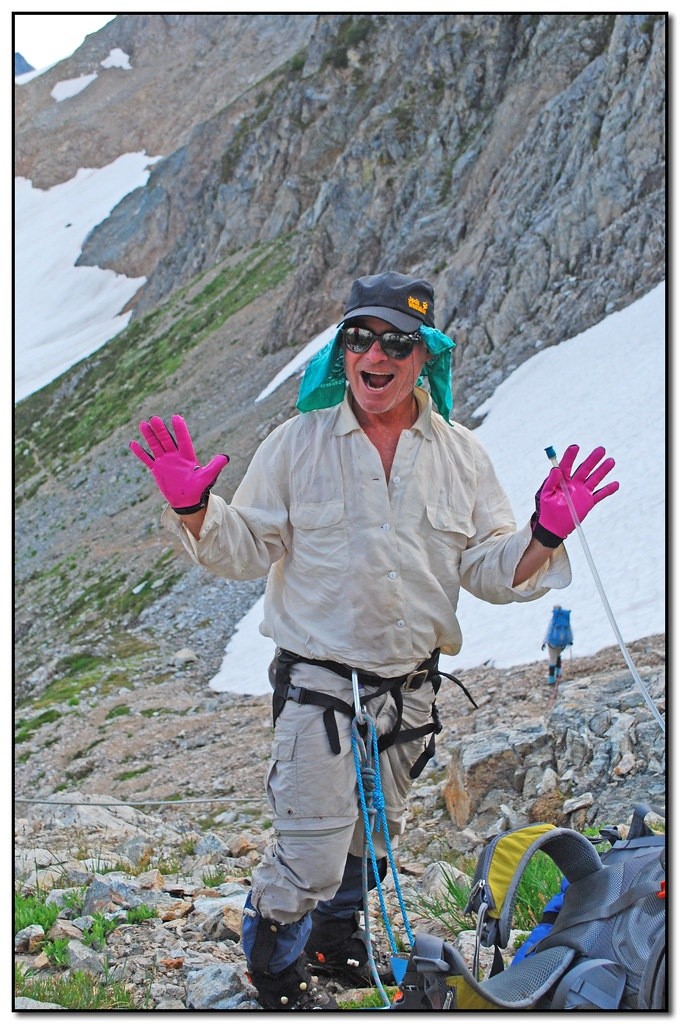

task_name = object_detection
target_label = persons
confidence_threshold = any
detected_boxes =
[128,274,620,1009]
[541,604,573,685]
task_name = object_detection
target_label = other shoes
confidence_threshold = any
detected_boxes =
[548,676,556,684]
[555,668,560,677]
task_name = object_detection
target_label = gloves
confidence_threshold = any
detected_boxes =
[530,445,619,547]
[541,644,545,650]
[129,414,231,515]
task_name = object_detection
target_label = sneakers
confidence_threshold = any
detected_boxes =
[261,978,341,1009]
[307,949,395,985]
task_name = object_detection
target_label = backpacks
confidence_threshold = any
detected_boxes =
[547,609,572,649]
[391,804,664,1009]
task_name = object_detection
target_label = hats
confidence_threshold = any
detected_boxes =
[338,271,435,333]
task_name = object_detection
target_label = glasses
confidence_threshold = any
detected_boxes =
[342,325,420,360]
